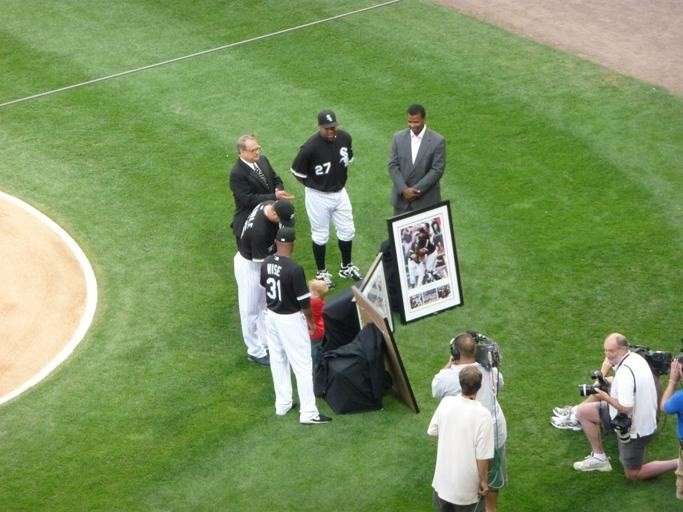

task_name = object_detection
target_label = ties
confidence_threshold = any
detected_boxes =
[252,162,269,188]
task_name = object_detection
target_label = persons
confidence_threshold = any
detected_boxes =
[661,355,683,502]
[230,135,294,250]
[402,219,446,286]
[291,110,363,288]
[261,228,332,424]
[431,333,507,512]
[573,333,679,479]
[388,104,447,214]
[234,198,296,366]
[307,279,328,396]
[427,366,494,512]
[549,357,663,432]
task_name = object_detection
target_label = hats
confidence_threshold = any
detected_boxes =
[276,226,298,243]
[316,109,342,129]
[314,270,337,290]
[338,263,367,281]
[273,195,298,228]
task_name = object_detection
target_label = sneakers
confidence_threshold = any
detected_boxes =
[246,348,271,368]
[571,452,613,474]
[549,403,580,433]
[301,411,336,426]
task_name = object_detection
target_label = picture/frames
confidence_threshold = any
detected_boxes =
[350,285,419,414]
[351,250,395,334]
[385,198,464,326]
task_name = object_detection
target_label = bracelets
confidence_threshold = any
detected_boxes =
[668,379,677,385]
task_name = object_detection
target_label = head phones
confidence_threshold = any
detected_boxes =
[449,333,467,360]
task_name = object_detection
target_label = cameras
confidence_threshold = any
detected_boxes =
[579,371,608,396]
[609,413,631,444]
